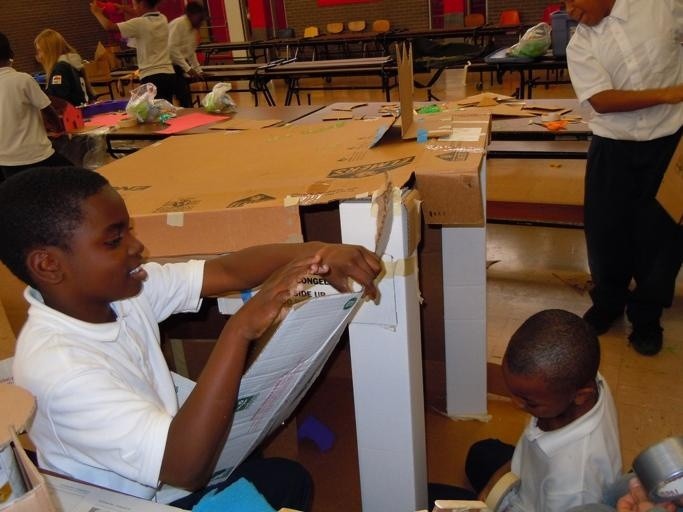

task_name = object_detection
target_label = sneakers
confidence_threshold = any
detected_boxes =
[625,297,662,355]
[583,302,624,335]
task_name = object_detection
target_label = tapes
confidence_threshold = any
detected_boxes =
[631,435,683,504]
[119,117,137,128]
[541,112,560,122]
[481,472,521,512]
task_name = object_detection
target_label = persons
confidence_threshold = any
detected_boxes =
[564,473,683,512]
[557,0,683,356]
[428,309,623,512]
[0,166,382,512]
[168,2,207,108]
[89,0,176,105]
[33,28,91,106]
[0,33,73,181]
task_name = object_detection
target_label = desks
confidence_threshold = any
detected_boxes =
[23,27,585,107]
[33,107,589,508]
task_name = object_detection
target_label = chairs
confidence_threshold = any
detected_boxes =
[462,2,562,29]
